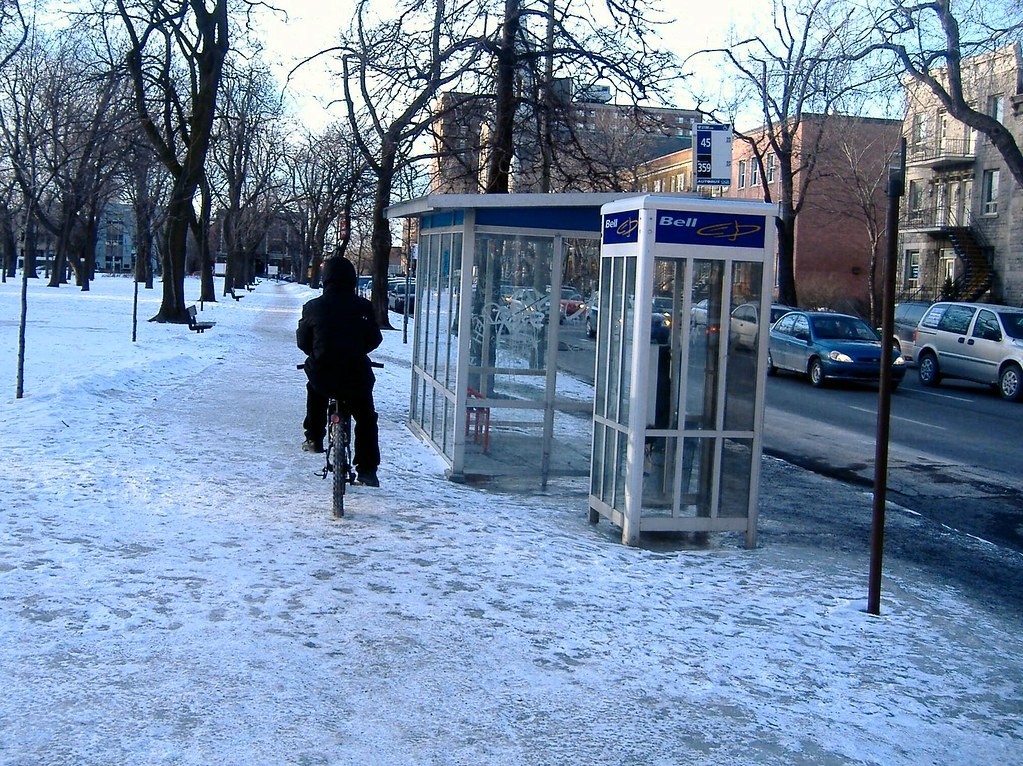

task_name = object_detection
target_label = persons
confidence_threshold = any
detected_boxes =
[295,255,384,488]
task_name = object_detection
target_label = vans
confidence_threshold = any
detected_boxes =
[911,302,1023,401]
[892,304,933,361]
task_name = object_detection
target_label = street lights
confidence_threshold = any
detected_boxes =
[105,240,118,275]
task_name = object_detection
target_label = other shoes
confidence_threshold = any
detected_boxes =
[306,437,324,453]
[358,470,380,488]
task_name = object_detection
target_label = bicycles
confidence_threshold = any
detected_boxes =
[295,362,385,519]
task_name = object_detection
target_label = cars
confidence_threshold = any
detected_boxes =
[355,275,416,314]
[767,312,907,393]
[691,298,707,328]
[585,291,672,344]
[546,285,586,316]
[731,301,792,352]
[500,285,564,326]
[652,297,683,324]
